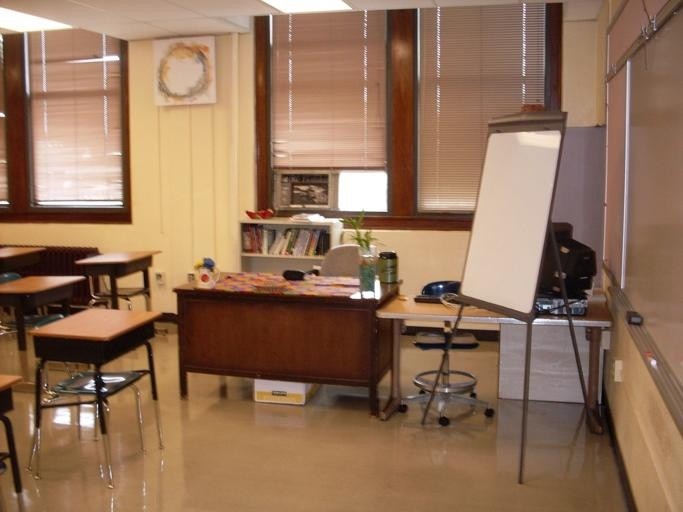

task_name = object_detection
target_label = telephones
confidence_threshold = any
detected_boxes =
[194,257,219,290]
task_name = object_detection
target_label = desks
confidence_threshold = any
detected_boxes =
[75,252,161,313]
[0,247,48,278]
[378,294,614,432]
[171,272,401,420]
[0,275,161,492]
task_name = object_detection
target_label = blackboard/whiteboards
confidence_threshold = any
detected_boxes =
[455,112,567,322]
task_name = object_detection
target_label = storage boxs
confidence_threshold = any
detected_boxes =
[252,375,323,404]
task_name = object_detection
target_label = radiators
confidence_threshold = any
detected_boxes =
[15,248,99,306]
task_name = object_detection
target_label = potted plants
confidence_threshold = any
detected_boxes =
[339,214,383,290]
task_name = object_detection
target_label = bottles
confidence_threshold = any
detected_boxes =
[358,254,376,292]
[379,252,398,285]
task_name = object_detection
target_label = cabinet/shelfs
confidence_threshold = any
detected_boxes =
[240,218,343,272]
[496,320,610,408]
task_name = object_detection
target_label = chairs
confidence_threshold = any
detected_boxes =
[403,281,496,430]
[320,244,378,277]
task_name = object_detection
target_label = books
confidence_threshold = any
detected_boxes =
[239,224,329,260]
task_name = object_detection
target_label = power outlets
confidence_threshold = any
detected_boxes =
[155,272,166,286]
[608,357,622,385]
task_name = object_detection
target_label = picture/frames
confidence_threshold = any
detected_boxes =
[273,166,339,211]
